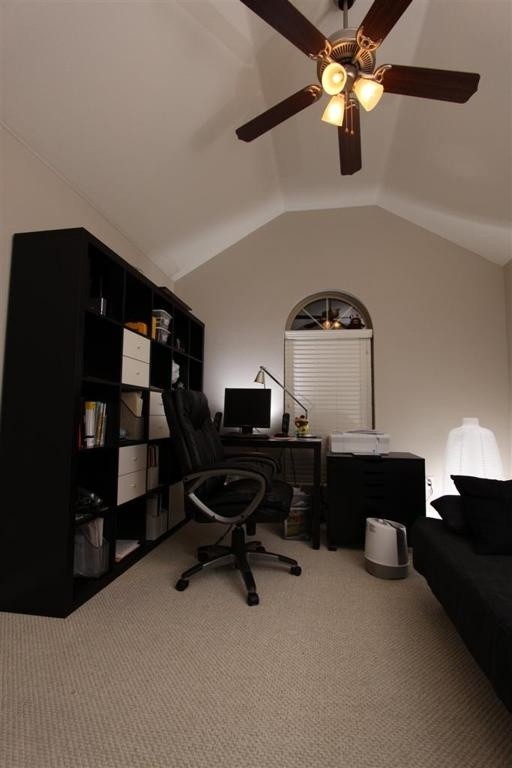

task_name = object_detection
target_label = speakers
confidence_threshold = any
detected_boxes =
[282,414,290,433]
[213,411,222,433]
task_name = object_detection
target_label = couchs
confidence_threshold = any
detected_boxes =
[411,515,512,715]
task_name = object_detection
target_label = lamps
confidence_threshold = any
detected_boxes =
[320,61,385,134]
[322,320,340,328]
[253,365,316,437]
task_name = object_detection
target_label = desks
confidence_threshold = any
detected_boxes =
[220,433,324,549]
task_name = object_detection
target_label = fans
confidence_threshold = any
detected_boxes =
[233,1,482,176]
[294,299,358,328]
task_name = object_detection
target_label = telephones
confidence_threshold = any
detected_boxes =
[274,413,291,437]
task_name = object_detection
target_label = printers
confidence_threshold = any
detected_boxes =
[329,430,390,456]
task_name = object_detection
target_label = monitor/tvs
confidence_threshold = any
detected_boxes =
[224,388,271,440]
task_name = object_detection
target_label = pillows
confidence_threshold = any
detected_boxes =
[431,474,512,555]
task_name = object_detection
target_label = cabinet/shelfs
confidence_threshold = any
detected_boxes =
[0,225,206,620]
[325,451,427,550]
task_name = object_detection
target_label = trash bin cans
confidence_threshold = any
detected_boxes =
[281,487,312,540]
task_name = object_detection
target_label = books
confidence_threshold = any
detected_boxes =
[79,401,109,446]
[115,539,140,561]
[146,493,162,519]
[147,443,159,468]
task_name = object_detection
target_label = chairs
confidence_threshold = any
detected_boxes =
[159,388,302,607]
